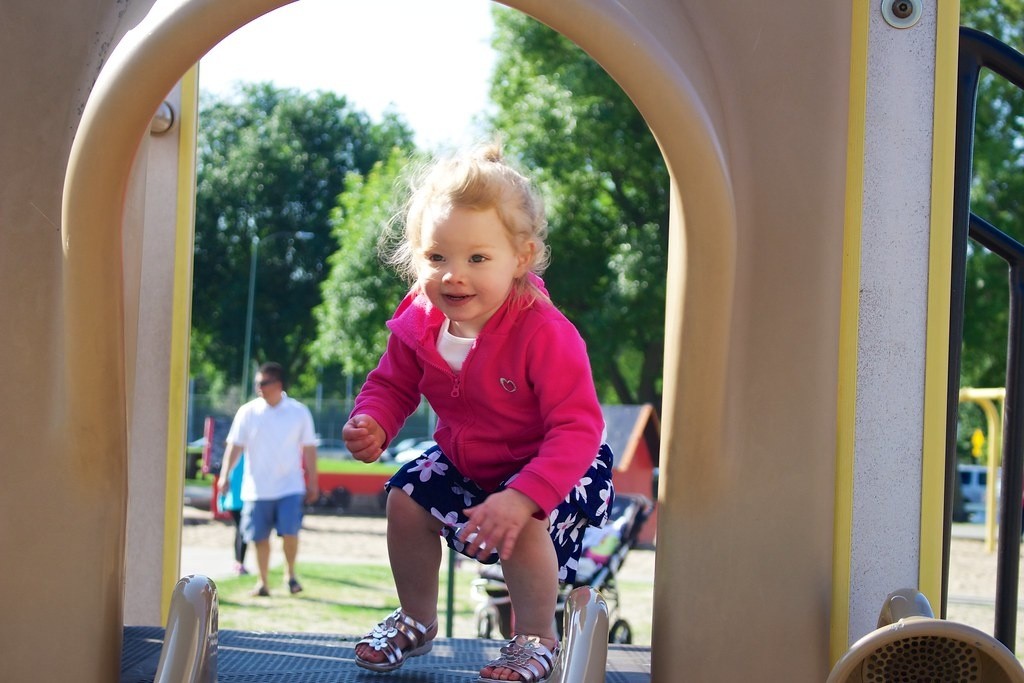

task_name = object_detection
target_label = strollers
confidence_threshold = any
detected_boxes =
[475,492,654,644]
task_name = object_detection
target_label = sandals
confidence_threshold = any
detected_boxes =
[477,634,560,683]
[355,607,438,671]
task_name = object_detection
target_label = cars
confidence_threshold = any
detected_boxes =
[958,464,1002,503]
[317,439,437,464]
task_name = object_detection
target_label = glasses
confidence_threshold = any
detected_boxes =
[256,379,273,387]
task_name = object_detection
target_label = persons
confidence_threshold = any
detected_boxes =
[216,362,321,596]
[343,145,615,683]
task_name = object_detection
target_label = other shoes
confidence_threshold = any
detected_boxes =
[238,565,247,576]
[258,585,268,596]
[287,578,302,593]
